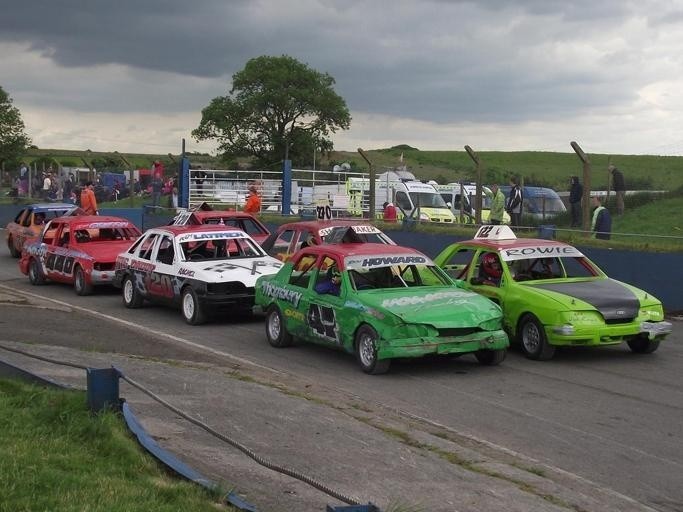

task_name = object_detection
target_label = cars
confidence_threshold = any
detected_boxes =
[171,209,290,255]
[8,202,100,257]
[434,238,673,361]
[261,220,398,270]
[115,224,285,325]
[18,215,144,296]
[255,242,510,375]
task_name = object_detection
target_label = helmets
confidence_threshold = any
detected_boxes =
[483,253,504,279]
[330,263,341,276]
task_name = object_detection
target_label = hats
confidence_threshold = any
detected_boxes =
[86,181,94,186]
[248,187,257,193]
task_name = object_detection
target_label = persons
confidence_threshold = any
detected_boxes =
[383,202,396,223]
[242,183,260,214]
[505,176,523,233]
[570,175,584,228]
[4,160,207,217]
[471,252,502,287]
[315,266,342,296]
[606,164,625,215]
[590,195,613,239]
[482,184,506,226]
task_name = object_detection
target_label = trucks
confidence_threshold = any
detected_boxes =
[346,176,456,225]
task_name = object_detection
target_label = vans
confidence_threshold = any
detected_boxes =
[433,184,511,224]
[489,184,566,225]
